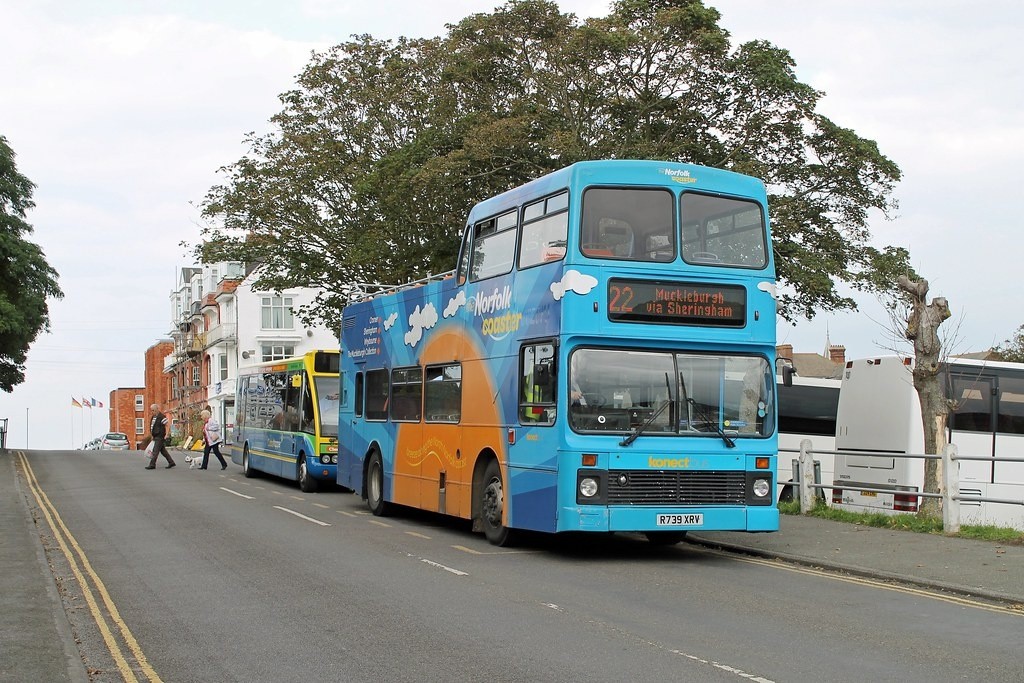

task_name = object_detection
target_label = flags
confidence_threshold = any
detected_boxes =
[92,398,103,407]
[83,397,91,409]
[72,397,82,408]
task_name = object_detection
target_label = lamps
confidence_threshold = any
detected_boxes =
[243,349,256,359]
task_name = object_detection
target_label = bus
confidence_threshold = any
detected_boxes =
[723,370,842,508]
[336,159,798,549]
[832,355,1024,532]
[230,348,341,492]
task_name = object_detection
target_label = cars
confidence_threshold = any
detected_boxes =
[98,433,130,451]
[76,437,101,451]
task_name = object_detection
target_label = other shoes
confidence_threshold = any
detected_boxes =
[165,463,176,468]
[221,464,227,470]
[144,465,155,469]
[198,466,207,470]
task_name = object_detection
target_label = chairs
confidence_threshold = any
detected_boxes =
[691,251,721,263]
[541,242,567,261]
[583,243,613,256]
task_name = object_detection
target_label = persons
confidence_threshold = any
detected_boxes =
[570,355,582,407]
[144,404,176,470]
[197,410,228,470]
[267,412,283,430]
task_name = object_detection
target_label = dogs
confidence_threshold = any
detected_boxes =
[185,456,204,469]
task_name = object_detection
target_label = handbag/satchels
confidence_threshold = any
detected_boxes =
[210,434,219,442]
[144,441,156,459]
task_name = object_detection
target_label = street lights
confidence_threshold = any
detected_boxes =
[26,408,29,449]
